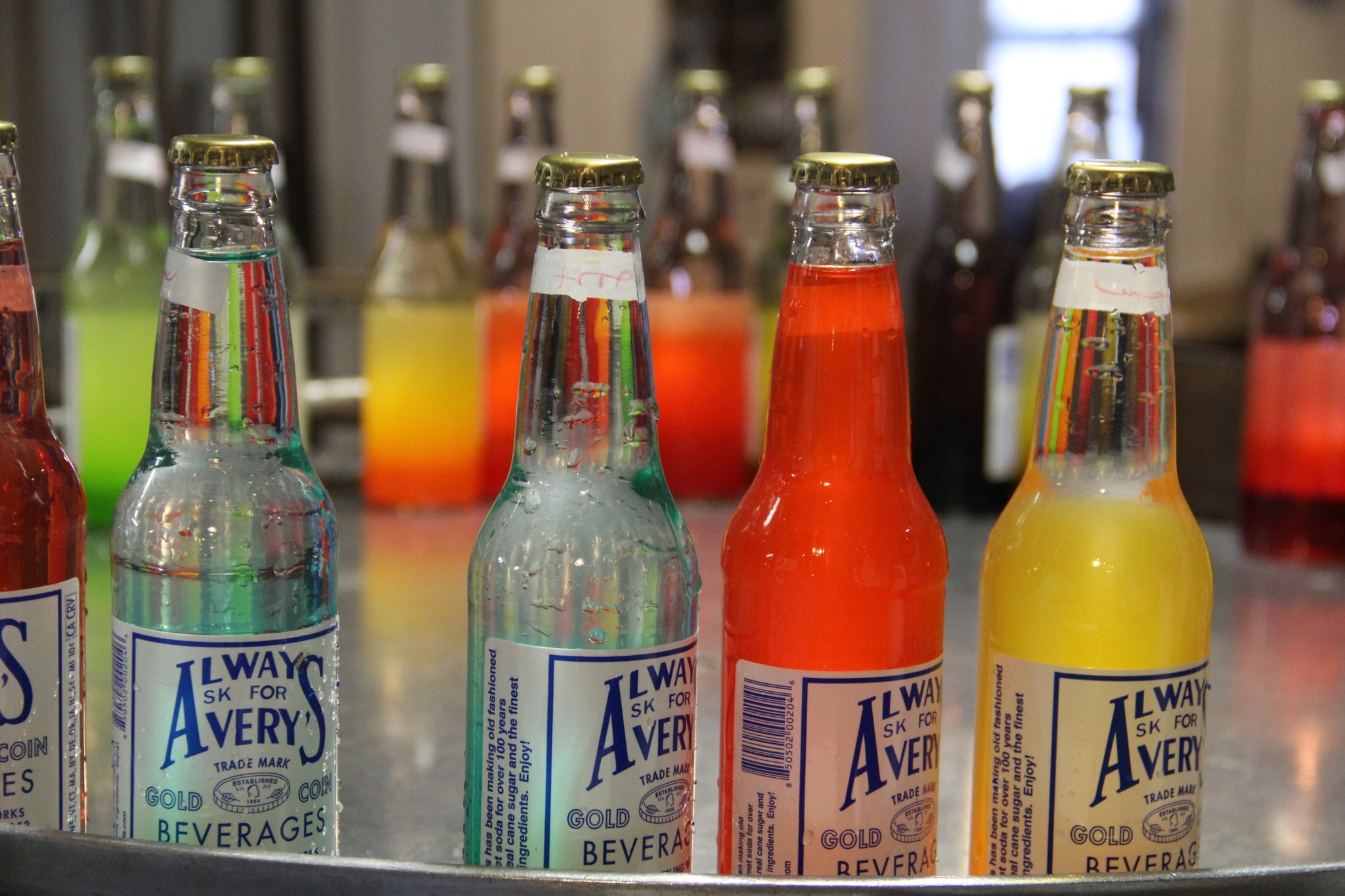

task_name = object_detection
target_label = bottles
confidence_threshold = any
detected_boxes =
[970,163,1214,879]
[1019,85,1109,465]
[478,66,556,507]
[466,156,697,877]
[748,68,836,485]
[1241,83,1343,570]
[361,66,485,509]
[641,71,756,504]
[61,56,173,521]
[0,122,85,840]
[717,151,948,879]
[212,59,305,451]
[112,134,339,857]
[904,71,1026,509]
